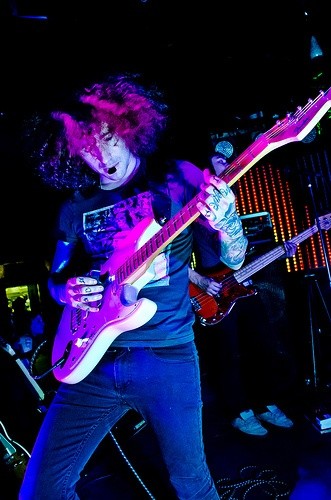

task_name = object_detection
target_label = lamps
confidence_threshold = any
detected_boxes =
[235,110,267,142]
[210,131,239,163]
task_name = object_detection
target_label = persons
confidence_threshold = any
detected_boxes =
[18,78,248,500]
[189,150,297,435]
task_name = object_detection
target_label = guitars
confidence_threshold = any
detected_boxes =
[189,212,331,327]
[48,85,331,386]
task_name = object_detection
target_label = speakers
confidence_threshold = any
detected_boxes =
[245,240,289,321]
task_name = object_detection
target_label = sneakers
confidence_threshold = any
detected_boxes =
[258,404,295,430]
[232,408,268,437]
